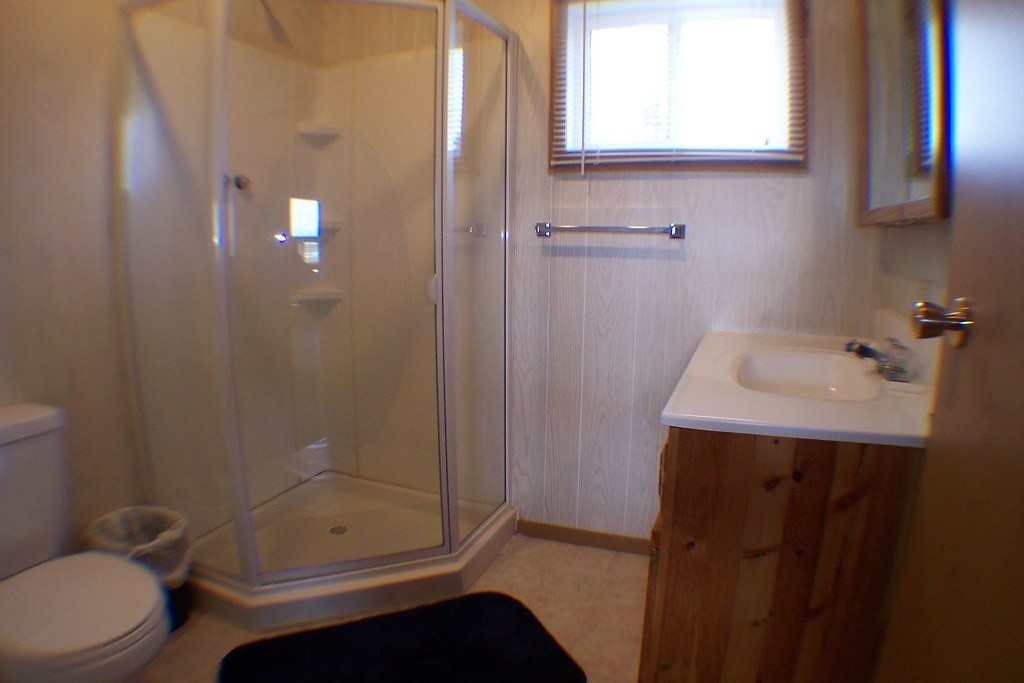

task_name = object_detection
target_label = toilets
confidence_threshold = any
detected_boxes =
[0,404,169,683]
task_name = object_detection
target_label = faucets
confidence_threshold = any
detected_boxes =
[844,341,888,361]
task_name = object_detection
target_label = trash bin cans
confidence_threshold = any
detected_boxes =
[83,505,194,632]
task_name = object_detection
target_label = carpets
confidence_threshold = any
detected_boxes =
[215,591,586,683]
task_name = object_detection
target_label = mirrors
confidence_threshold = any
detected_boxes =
[855,0,951,228]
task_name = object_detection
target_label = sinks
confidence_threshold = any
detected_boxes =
[659,328,929,449]
